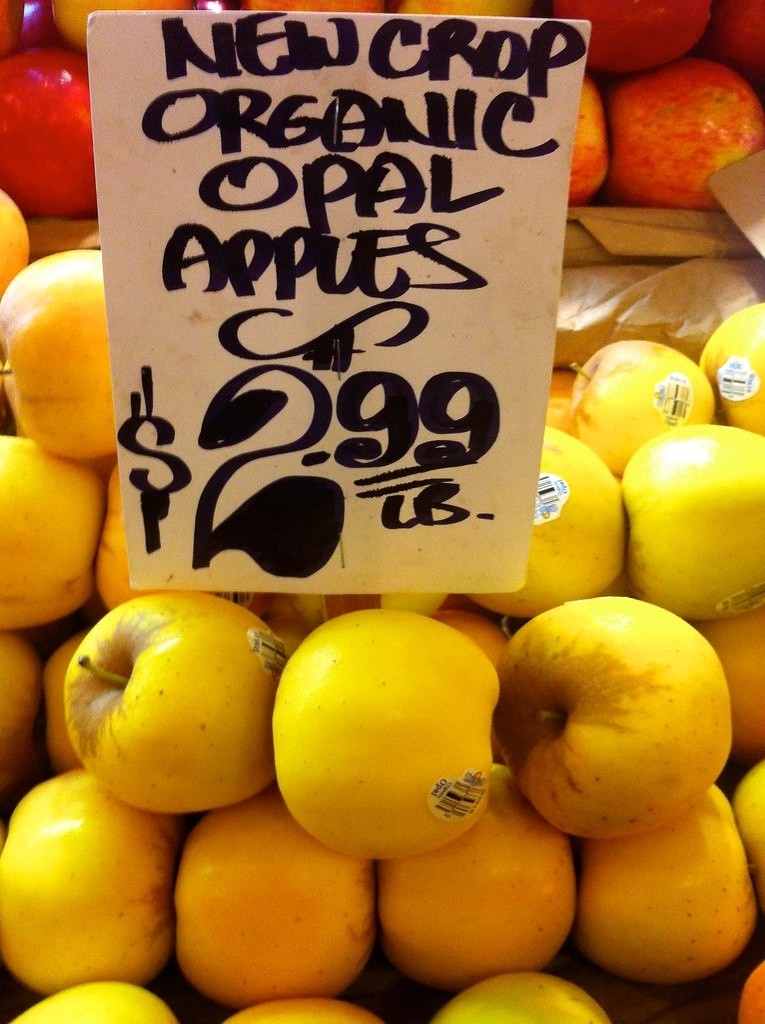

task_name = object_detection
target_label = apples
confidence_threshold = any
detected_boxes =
[0,0,765,1024]
